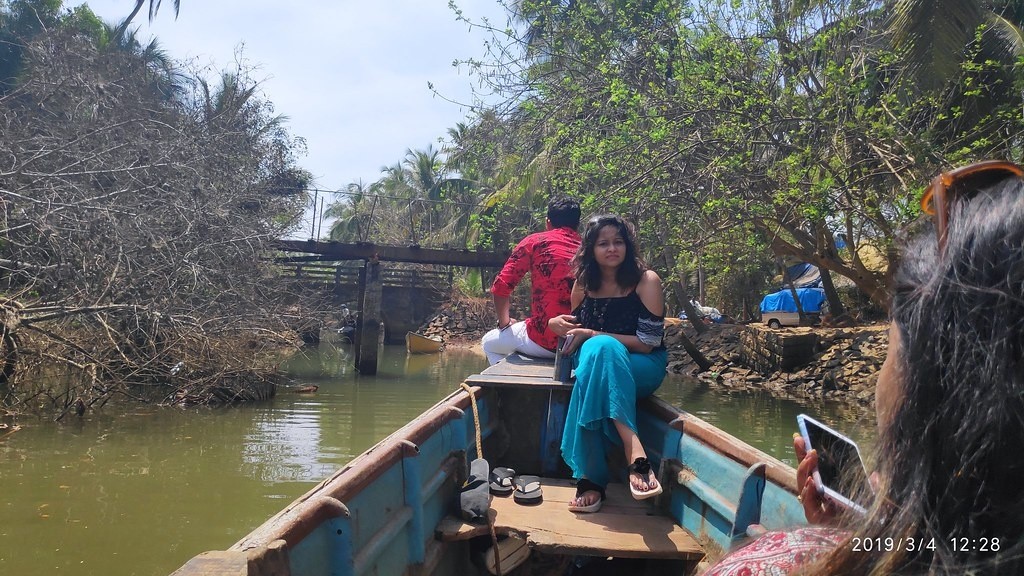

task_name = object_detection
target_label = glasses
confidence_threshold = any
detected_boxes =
[921,160,1024,271]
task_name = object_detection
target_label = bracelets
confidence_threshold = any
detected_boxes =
[496,318,513,331]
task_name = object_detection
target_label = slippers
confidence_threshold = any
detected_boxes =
[491,466,516,495]
[627,456,663,500]
[512,475,542,504]
[567,474,605,513]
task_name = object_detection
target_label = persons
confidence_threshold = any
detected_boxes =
[481,195,583,366]
[695,170,1024,576]
[545,214,668,515]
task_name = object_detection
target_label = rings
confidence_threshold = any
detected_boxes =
[796,494,804,504]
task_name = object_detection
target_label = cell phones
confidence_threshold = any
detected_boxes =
[797,414,876,515]
[560,334,575,352]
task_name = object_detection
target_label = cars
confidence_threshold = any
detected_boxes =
[761,288,828,329]
[679,300,720,320]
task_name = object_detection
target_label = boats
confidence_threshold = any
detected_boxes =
[170,352,906,575]
[406,330,444,354]
